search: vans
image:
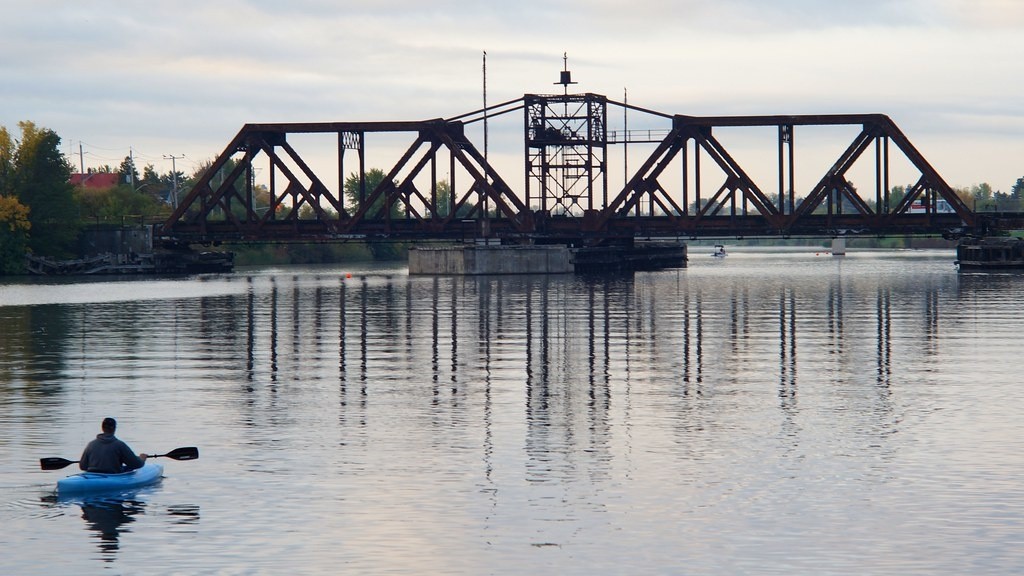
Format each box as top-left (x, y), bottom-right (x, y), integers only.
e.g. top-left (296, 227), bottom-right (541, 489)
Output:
top-left (908, 200), bottom-right (956, 213)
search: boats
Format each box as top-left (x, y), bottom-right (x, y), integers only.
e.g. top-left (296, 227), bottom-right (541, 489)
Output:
top-left (714, 244), bottom-right (727, 258)
top-left (56, 459), bottom-right (165, 500)
top-left (197, 250), bottom-right (235, 273)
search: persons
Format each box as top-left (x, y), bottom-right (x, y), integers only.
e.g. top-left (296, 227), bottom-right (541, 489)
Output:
top-left (79, 418), bottom-right (147, 474)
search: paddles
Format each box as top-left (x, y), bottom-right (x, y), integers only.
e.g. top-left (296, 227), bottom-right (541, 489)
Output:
top-left (39, 446), bottom-right (200, 471)
